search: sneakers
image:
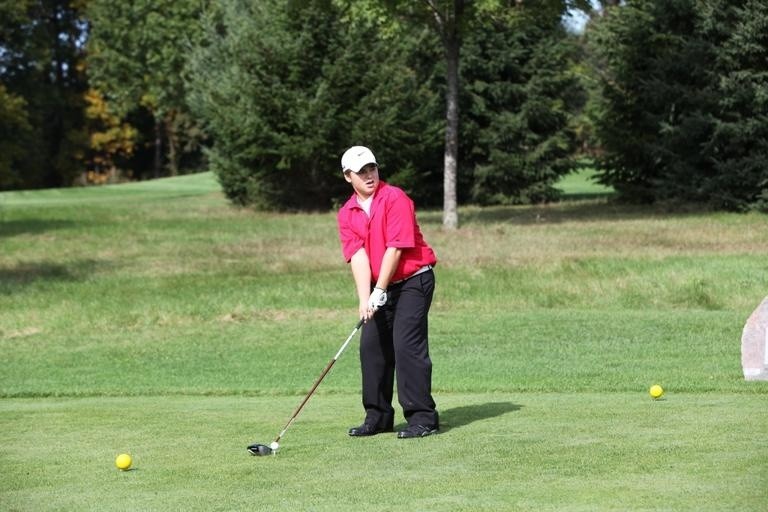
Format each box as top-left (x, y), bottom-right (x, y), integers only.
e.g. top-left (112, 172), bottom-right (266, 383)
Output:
top-left (397, 424), bottom-right (439, 439)
top-left (349, 423), bottom-right (394, 436)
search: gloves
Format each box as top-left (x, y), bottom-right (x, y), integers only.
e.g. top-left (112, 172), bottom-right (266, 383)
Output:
top-left (368, 288), bottom-right (384, 310)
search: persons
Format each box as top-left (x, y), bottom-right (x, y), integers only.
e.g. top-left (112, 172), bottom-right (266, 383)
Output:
top-left (336, 144), bottom-right (441, 439)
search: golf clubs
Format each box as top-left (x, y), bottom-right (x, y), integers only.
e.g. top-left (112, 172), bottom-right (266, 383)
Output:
top-left (246, 290), bottom-right (387, 456)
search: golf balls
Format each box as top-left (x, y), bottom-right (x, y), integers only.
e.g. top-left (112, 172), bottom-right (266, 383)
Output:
top-left (270, 441), bottom-right (279, 450)
top-left (649, 385), bottom-right (663, 400)
top-left (116, 453), bottom-right (132, 470)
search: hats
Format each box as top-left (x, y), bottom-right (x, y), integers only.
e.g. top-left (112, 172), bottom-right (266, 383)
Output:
top-left (341, 146), bottom-right (379, 173)
